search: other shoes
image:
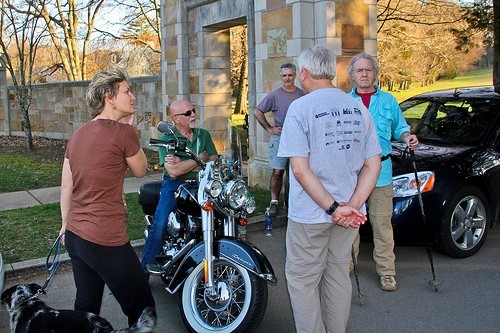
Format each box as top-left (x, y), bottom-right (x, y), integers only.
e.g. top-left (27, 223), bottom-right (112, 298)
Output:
top-left (379, 274), bottom-right (398, 292)
top-left (269, 200), bottom-right (279, 215)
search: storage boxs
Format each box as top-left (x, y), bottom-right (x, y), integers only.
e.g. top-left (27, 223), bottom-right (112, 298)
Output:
top-left (139, 181), bottom-right (161, 215)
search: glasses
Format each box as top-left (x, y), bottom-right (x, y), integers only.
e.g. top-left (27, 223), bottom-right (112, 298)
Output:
top-left (172, 108), bottom-right (197, 119)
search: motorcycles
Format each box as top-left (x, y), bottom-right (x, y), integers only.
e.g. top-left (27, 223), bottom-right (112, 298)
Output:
top-left (138, 121), bottom-right (278, 333)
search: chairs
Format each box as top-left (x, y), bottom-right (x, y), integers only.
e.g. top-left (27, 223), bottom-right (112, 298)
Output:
top-left (434, 108), bottom-right (471, 138)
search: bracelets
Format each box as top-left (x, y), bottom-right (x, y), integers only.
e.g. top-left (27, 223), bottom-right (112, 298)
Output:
top-left (325, 201), bottom-right (339, 215)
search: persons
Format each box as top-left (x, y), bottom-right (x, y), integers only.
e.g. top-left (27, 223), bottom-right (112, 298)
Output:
top-left (59, 72), bottom-right (156, 333)
top-left (230, 63), bottom-right (306, 217)
top-left (277, 45), bottom-right (382, 333)
top-left (140, 100), bottom-right (219, 277)
top-left (347, 54), bottom-right (418, 291)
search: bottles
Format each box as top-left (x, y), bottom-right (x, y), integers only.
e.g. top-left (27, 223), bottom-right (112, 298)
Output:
top-left (265, 207), bottom-right (273, 237)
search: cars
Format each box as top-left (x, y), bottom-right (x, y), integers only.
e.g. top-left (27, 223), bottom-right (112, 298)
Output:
top-left (358, 85), bottom-right (500, 260)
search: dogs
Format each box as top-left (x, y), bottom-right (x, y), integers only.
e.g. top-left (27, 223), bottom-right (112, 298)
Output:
top-left (0, 283), bottom-right (155, 333)
